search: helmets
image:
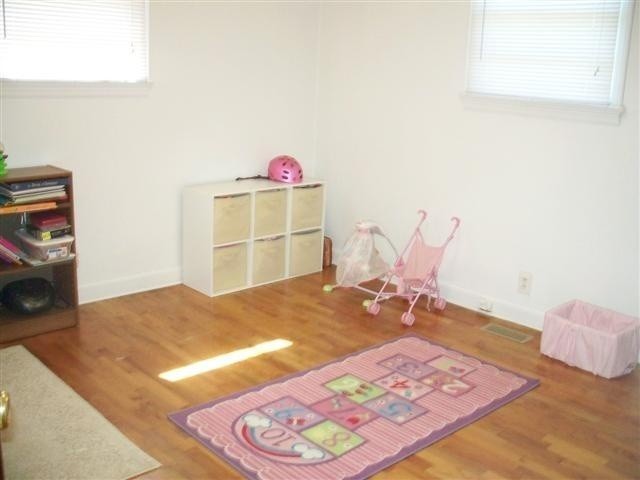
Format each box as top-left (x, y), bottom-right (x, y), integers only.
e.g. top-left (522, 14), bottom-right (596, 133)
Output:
top-left (268, 156), bottom-right (303, 183)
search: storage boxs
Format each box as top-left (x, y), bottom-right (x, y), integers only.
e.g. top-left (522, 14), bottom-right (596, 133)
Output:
top-left (538, 297), bottom-right (640, 381)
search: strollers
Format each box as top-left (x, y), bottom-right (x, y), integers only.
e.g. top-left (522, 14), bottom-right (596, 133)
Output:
top-left (366, 209), bottom-right (461, 327)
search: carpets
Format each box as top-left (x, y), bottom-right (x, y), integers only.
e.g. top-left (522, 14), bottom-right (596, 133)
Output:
top-left (0, 344), bottom-right (164, 480)
top-left (166, 331), bottom-right (543, 479)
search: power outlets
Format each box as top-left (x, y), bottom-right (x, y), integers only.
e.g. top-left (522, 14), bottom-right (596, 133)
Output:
top-left (515, 269), bottom-right (534, 298)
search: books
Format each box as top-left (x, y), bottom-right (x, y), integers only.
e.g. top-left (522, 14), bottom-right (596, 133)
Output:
top-left (1, 177), bottom-right (75, 265)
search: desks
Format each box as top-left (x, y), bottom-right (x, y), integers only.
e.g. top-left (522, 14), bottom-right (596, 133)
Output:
top-left (180, 177), bottom-right (327, 298)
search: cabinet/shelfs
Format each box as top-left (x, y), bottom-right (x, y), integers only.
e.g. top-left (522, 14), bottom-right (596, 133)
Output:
top-left (0, 163), bottom-right (86, 346)
